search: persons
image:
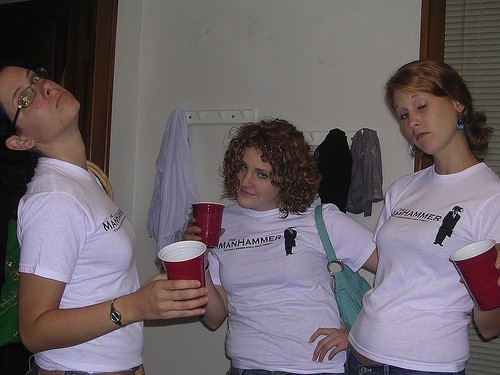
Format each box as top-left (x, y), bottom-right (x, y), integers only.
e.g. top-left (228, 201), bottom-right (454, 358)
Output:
top-left (0, 65), bottom-right (209, 374)
top-left (344, 60), bottom-right (500, 375)
top-left (182, 118), bottom-right (381, 375)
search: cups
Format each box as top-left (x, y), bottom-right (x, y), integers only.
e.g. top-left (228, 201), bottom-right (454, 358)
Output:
top-left (191, 201), bottom-right (226, 249)
top-left (449, 239), bottom-right (500, 310)
top-left (157, 240), bottom-right (206, 309)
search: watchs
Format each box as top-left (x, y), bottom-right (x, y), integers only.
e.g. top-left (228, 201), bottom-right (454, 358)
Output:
top-left (110, 297), bottom-right (125, 328)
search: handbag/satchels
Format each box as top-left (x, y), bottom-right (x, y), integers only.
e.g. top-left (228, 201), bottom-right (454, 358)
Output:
top-left (315, 204), bottom-right (373, 330)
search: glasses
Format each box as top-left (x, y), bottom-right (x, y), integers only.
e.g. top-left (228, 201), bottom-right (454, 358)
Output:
top-left (13, 66), bottom-right (47, 125)
